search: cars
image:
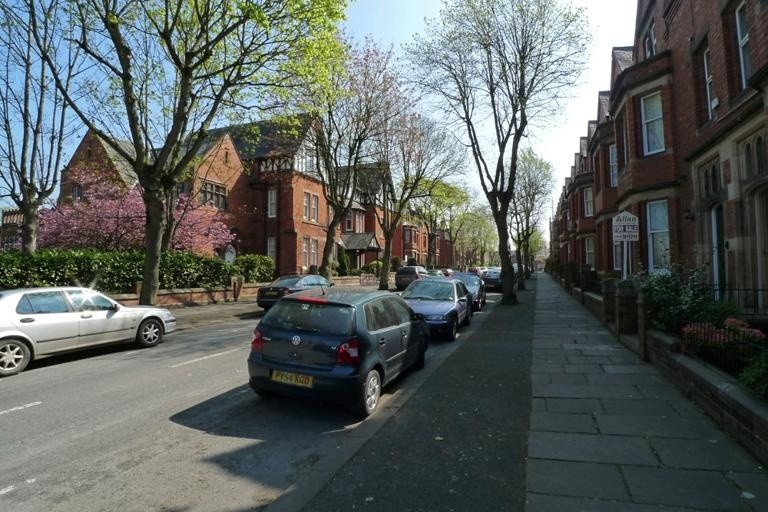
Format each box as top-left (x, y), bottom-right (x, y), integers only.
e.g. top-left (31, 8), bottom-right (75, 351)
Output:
top-left (471, 263), bottom-right (526, 290)
top-left (246, 288), bottom-right (430, 415)
top-left (0, 285), bottom-right (176, 377)
top-left (449, 272), bottom-right (486, 311)
top-left (256, 274), bottom-right (327, 311)
top-left (402, 277), bottom-right (473, 341)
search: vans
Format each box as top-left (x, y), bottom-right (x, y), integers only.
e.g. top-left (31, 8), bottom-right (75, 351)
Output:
top-left (394, 265), bottom-right (451, 288)
